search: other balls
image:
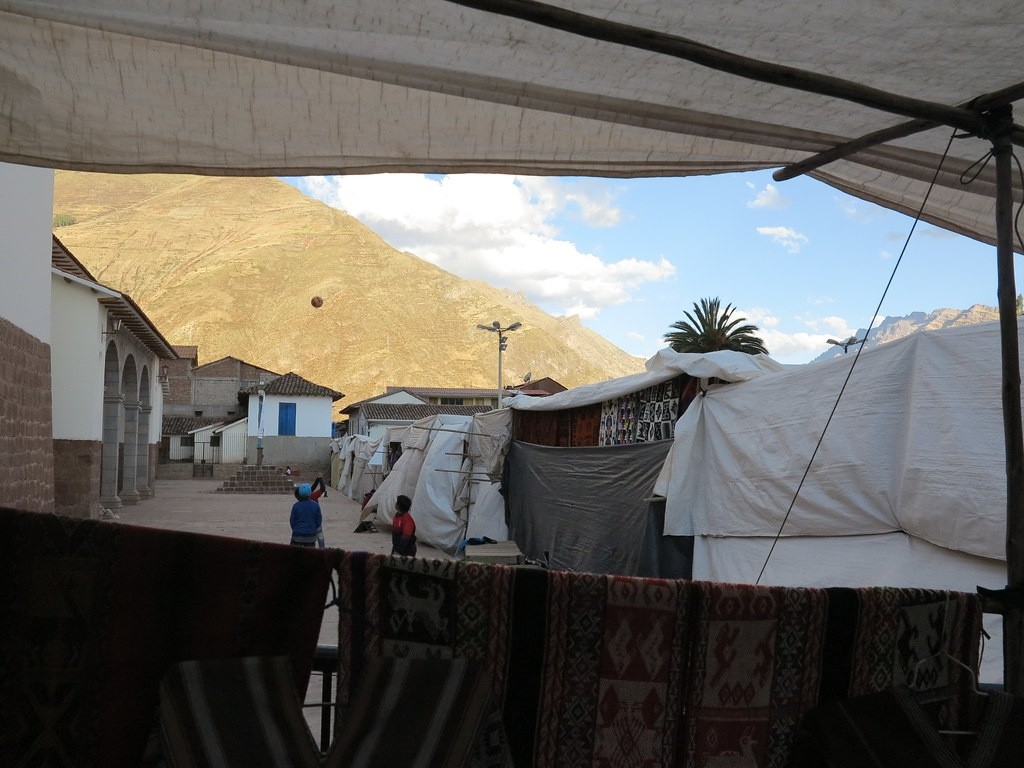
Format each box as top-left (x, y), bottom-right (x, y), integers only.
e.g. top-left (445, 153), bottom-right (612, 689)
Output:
top-left (311, 297), bottom-right (323, 309)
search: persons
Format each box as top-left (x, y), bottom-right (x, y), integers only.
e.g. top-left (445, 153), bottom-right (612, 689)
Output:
top-left (391, 495), bottom-right (417, 557)
top-left (289, 472), bottom-right (327, 548)
top-left (361, 489), bottom-right (378, 513)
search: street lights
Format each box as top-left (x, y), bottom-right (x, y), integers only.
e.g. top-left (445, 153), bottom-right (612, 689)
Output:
top-left (476, 320), bottom-right (522, 408)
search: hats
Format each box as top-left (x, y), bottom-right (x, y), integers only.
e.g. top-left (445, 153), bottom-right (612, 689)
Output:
top-left (299, 483), bottom-right (311, 497)
